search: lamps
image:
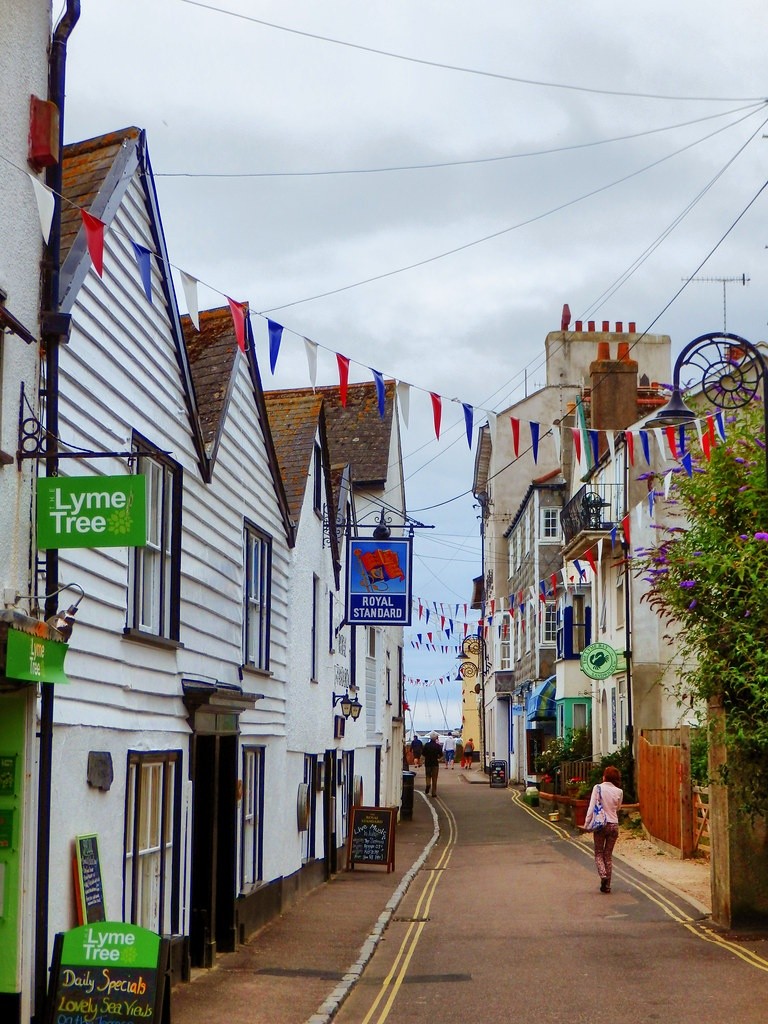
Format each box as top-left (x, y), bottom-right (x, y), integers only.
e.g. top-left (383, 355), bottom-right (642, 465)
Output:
top-left (519, 682), bottom-right (531, 697)
top-left (454, 662), bottom-right (478, 682)
top-left (3, 583), bottom-right (85, 639)
top-left (371, 509), bottom-right (391, 540)
top-left (333, 688), bottom-right (362, 722)
top-left (641, 330), bottom-right (768, 485)
top-left (455, 633), bottom-right (488, 661)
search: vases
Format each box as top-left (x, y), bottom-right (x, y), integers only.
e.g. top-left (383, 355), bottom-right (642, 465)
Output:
top-left (568, 785), bottom-right (579, 795)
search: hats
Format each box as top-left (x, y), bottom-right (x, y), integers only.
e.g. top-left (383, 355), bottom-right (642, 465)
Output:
top-left (430, 733), bottom-right (440, 740)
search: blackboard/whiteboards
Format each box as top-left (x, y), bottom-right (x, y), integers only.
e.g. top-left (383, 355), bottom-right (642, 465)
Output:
top-left (346, 805), bottom-right (396, 865)
top-left (76, 833), bottom-right (106, 925)
top-left (42, 922), bottom-right (173, 1024)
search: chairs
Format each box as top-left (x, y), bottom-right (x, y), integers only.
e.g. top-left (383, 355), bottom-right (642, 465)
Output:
top-left (580, 492), bottom-right (603, 529)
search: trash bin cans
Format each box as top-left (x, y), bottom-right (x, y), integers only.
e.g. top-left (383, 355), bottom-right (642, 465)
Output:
top-left (400, 771), bottom-right (416, 820)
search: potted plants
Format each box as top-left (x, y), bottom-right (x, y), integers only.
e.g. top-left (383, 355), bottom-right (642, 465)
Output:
top-left (575, 783), bottom-right (592, 799)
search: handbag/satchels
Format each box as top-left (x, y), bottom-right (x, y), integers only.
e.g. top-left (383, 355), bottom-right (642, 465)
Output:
top-left (461, 754), bottom-right (465, 767)
top-left (592, 784), bottom-right (607, 831)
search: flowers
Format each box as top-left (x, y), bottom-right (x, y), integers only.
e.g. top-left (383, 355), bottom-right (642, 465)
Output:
top-left (566, 776), bottom-right (587, 786)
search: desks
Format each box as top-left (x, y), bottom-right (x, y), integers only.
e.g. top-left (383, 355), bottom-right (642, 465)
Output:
top-left (590, 502), bottom-right (611, 527)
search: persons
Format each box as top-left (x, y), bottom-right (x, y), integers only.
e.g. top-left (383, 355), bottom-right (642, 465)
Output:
top-left (410, 736), bottom-right (423, 768)
top-left (583, 767), bottom-right (623, 894)
top-left (443, 732), bottom-right (457, 770)
top-left (463, 738), bottom-right (475, 770)
top-left (422, 732), bottom-right (443, 799)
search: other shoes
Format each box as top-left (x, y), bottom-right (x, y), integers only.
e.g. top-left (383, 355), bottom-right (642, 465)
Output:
top-left (600, 879), bottom-right (607, 892)
top-left (605, 888), bottom-right (610, 893)
top-left (432, 794), bottom-right (437, 798)
top-left (425, 785), bottom-right (431, 794)
